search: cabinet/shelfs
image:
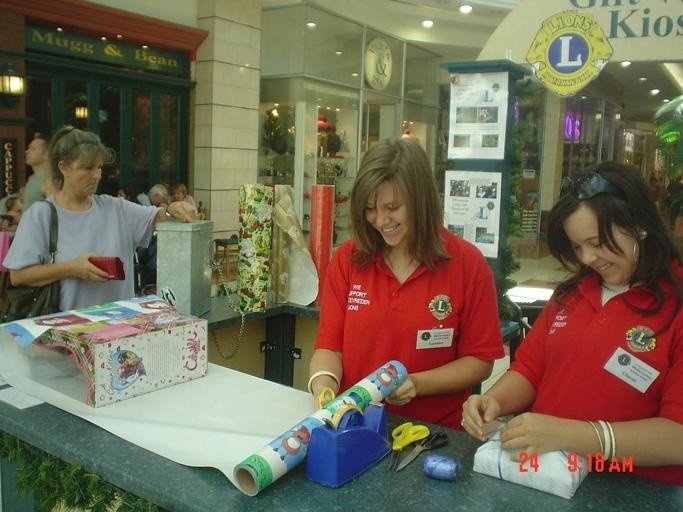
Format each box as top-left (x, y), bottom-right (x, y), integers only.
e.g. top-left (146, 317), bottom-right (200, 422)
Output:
top-left (506, 84), bottom-right (652, 260)
top-left (257, 75), bottom-right (442, 303)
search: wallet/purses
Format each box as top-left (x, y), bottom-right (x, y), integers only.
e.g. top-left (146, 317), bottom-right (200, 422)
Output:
top-left (88, 256), bottom-right (126, 280)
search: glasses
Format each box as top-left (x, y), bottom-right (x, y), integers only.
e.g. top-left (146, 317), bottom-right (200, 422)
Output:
top-left (560, 172), bottom-right (624, 200)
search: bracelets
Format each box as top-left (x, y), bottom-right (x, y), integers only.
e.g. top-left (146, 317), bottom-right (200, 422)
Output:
top-left (597, 419), bottom-right (610, 461)
top-left (606, 421), bottom-right (616, 462)
top-left (586, 419), bottom-right (605, 454)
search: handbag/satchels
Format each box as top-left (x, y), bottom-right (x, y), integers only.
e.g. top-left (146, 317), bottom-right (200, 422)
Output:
top-left (0, 200), bottom-right (59, 324)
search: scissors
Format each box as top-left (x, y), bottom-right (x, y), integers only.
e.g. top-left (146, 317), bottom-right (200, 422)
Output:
top-left (319, 388), bottom-right (335, 409)
top-left (386, 422), bottom-right (448, 472)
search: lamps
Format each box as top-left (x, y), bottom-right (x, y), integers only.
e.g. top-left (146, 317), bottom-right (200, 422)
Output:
top-left (0, 61), bottom-right (25, 109)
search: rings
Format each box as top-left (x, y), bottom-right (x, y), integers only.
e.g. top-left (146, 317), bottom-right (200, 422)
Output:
top-left (460, 418), bottom-right (465, 426)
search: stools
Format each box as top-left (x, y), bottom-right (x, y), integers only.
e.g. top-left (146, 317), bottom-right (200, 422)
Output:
top-left (214, 239), bottom-right (240, 281)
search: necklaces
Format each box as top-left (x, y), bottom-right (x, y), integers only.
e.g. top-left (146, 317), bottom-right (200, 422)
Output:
top-left (307, 370), bottom-right (340, 395)
top-left (598, 283), bottom-right (618, 294)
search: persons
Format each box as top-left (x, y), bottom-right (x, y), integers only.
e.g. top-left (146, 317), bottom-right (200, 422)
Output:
top-left (1, 125), bottom-right (199, 311)
top-left (20, 132), bottom-right (51, 213)
top-left (0, 182), bottom-right (199, 287)
top-left (461, 161), bottom-right (683, 487)
top-left (648, 175), bottom-right (683, 232)
top-left (306, 134), bottom-right (506, 431)
